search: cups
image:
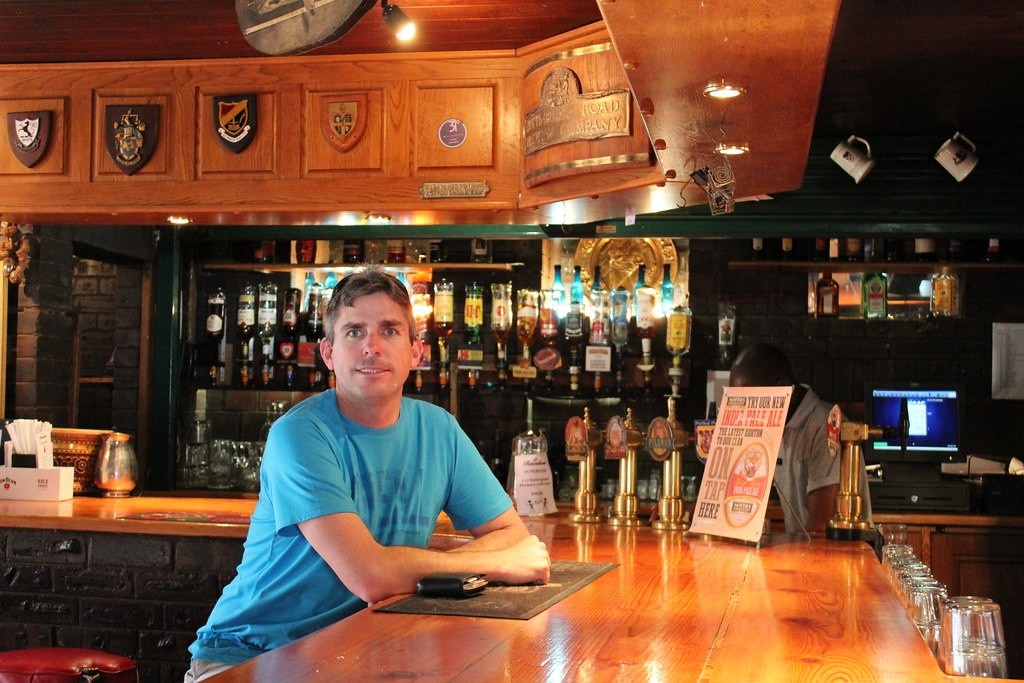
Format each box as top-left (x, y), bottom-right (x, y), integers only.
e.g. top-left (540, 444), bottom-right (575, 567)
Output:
top-left (174, 400), bottom-right (291, 492)
top-left (879, 523), bottom-right (1009, 679)
top-left (830, 135), bottom-right (878, 183)
top-left (934, 131), bottom-right (979, 182)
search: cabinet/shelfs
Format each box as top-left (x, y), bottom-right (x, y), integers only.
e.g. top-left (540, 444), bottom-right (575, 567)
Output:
top-left (923, 516), bottom-right (1024, 646)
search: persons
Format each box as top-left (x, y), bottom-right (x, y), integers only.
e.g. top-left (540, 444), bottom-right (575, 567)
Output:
top-left (184, 271), bottom-right (551, 683)
top-left (731, 346), bottom-right (874, 534)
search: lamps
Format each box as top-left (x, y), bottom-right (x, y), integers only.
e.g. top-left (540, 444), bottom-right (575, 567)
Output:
top-left (381, 1), bottom-right (418, 43)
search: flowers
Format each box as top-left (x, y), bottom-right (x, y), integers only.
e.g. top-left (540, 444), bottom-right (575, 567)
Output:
top-left (0, 223), bottom-right (34, 284)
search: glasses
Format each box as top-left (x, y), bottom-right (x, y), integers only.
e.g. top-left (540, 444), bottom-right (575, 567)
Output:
top-left (331, 272), bottom-right (408, 295)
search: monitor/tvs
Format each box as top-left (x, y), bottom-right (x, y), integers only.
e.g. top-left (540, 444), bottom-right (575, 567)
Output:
top-left (870, 387), bottom-right (966, 464)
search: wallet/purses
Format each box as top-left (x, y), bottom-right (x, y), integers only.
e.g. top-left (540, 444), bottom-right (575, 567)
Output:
top-left (417, 571), bottom-right (487, 598)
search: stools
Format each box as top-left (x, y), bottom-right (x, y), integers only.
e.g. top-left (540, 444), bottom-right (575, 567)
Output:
top-left (1, 649), bottom-right (140, 683)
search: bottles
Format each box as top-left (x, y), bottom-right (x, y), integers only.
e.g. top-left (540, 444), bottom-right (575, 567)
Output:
top-left (206, 240), bottom-right (692, 394)
top-left (716, 295), bottom-right (737, 371)
top-left (489, 428), bottom-right (505, 487)
top-left (601, 478), bottom-right (617, 499)
top-left (752, 238), bottom-right (1003, 319)
top-left (680, 475), bottom-right (697, 502)
top-left (648, 468), bottom-right (662, 501)
